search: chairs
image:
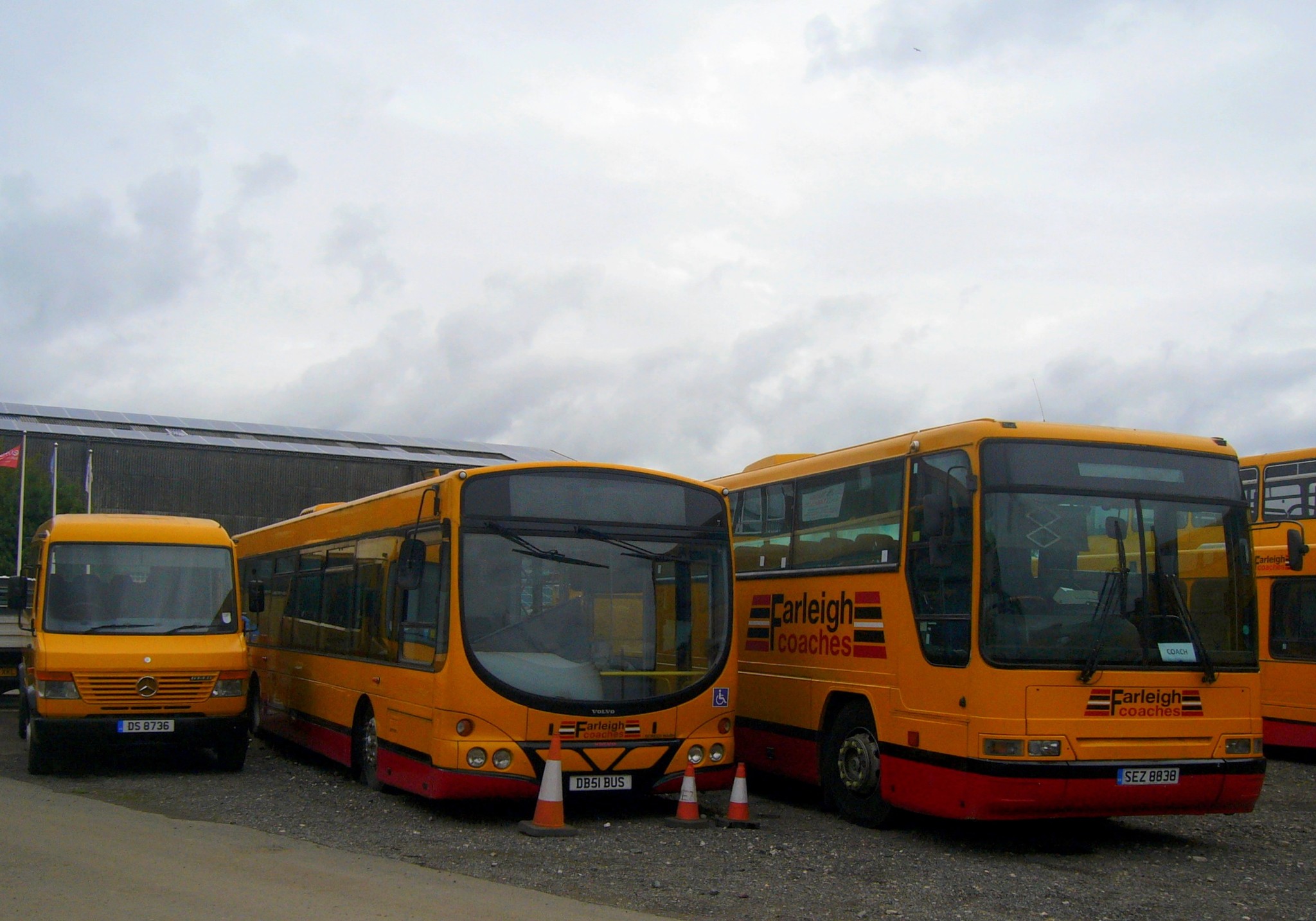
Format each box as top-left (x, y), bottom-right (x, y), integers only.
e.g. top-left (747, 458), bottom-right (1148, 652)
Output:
top-left (734, 515), bottom-right (1098, 657)
top-left (48, 566), bottom-right (204, 621)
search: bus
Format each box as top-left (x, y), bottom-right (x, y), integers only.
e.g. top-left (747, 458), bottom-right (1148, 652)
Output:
top-left (1020, 446), bottom-right (1316, 749)
top-left (230, 462), bottom-right (739, 809)
top-left (704, 419), bottom-right (1265, 843)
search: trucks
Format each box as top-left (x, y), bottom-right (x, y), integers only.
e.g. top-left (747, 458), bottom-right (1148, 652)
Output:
top-left (0, 575), bottom-right (39, 710)
top-left (6, 512), bottom-right (268, 778)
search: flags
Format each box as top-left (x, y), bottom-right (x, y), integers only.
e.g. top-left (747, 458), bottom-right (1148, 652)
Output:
top-left (0, 442), bottom-right (21, 470)
top-left (50, 453), bottom-right (55, 484)
top-left (85, 457), bottom-right (94, 492)
